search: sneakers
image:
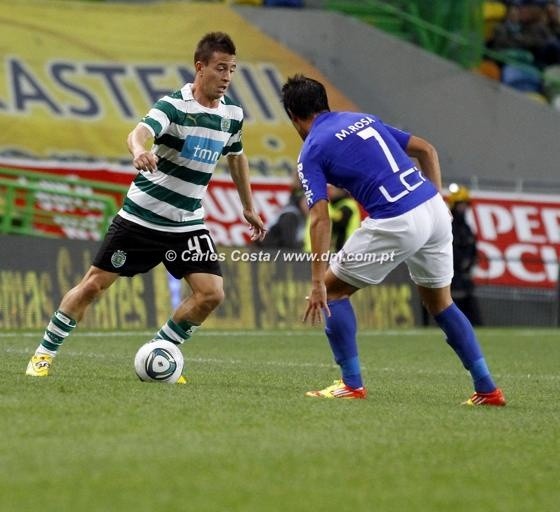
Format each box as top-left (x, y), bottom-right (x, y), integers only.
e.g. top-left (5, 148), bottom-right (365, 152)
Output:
top-left (304, 377), bottom-right (365, 398)
top-left (25, 351), bottom-right (55, 378)
top-left (461, 389), bottom-right (508, 408)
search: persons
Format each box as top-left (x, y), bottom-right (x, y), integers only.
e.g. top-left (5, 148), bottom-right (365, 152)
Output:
top-left (256, 176), bottom-right (485, 327)
top-left (24, 32), bottom-right (269, 384)
top-left (474, 0), bottom-right (560, 110)
top-left (281, 75), bottom-right (505, 406)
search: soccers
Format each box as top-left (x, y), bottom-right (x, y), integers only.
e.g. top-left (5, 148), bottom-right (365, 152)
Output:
top-left (135, 339), bottom-right (184, 384)
